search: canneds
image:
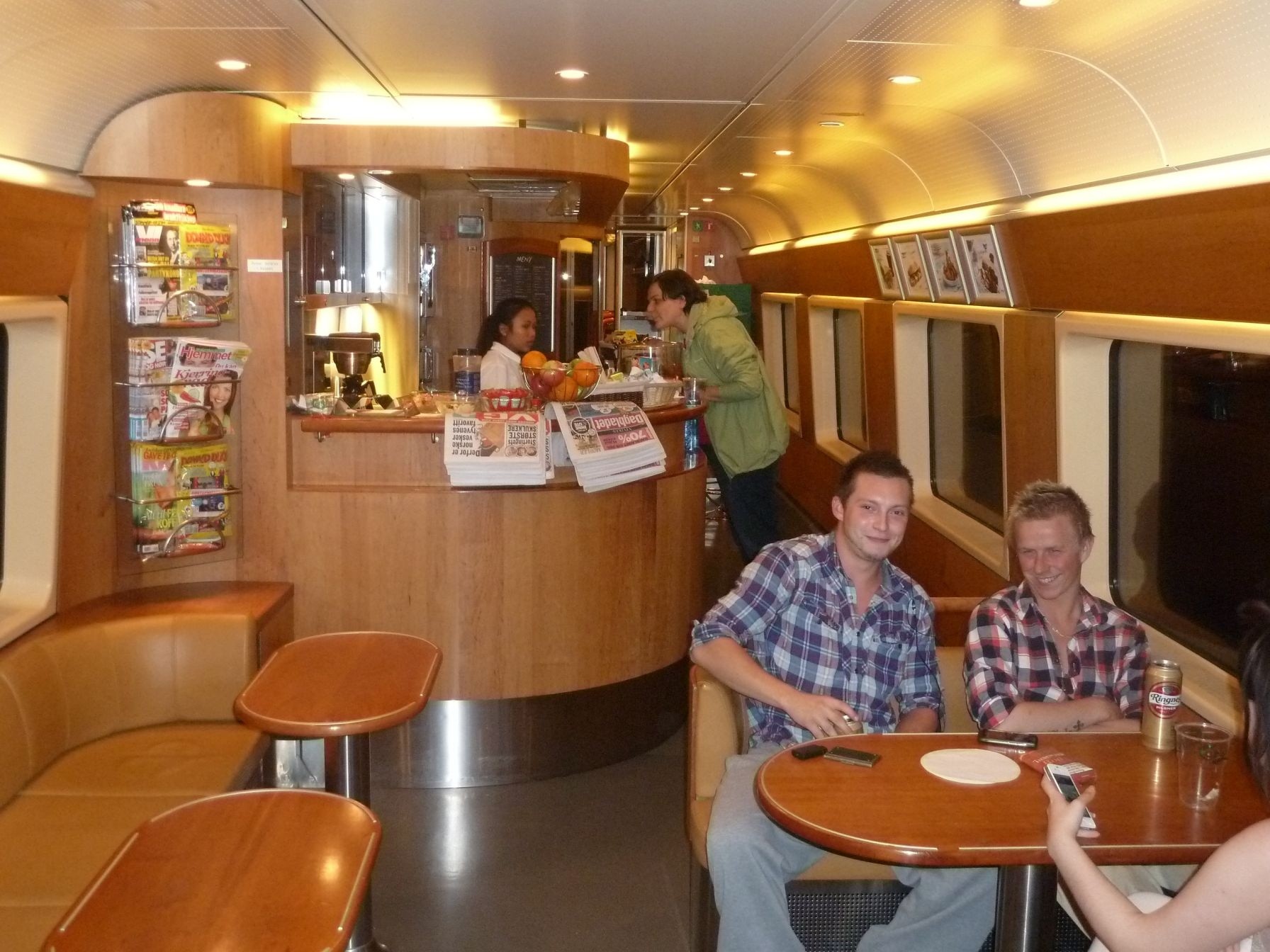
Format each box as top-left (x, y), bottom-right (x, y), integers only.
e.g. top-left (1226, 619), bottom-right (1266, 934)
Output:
top-left (1139, 658), bottom-right (1186, 754)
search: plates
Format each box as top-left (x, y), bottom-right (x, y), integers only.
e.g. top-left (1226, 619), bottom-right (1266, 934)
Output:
top-left (920, 748), bottom-right (1021, 785)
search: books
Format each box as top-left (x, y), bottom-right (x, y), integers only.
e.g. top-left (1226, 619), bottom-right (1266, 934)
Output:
top-left (122, 218), bottom-right (252, 558)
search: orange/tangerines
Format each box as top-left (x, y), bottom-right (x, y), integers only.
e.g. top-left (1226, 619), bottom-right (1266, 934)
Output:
top-left (522, 351), bottom-right (547, 369)
top-left (551, 377), bottom-right (579, 402)
top-left (573, 362), bottom-right (600, 387)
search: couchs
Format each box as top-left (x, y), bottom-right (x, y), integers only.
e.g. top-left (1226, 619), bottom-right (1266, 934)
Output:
top-left (684, 594), bottom-right (988, 952)
top-left (0, 579), bottom-right (295, 952)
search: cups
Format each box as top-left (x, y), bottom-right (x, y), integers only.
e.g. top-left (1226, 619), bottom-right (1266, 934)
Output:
top-left (1175, 723), bottom-right (1231, 805)
top-left (681, 378), bottom-right (707, 407)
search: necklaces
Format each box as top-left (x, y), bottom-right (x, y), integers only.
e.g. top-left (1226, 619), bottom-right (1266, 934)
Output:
top-left (1038, 608), bottom-right (1075, 639)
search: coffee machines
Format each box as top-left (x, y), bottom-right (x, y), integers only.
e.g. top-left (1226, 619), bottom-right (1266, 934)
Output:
top-left (304, 330), bottom-right (385, 399)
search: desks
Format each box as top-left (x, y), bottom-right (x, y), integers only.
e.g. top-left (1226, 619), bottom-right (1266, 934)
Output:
top-left (753, 732), bottom-right (1270, 952)
top-left (39, 787), bottom-right (383, 952)
top-left (232, 629), bottom-right (442, 952)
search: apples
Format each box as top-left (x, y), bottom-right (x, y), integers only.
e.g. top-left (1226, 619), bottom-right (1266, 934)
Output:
top-left (540, 360), bottom-right (567, 386)
top-left (529, 376), bottom-right (552, 400)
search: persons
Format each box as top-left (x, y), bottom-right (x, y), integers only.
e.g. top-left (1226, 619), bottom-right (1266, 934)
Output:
top-left (647, 268), bottom-right (789, 564)
top-left (1038, 604), bottom-right (1270, 951)
top-left (477, 297), bottom-right (537, 391)
top-left (962, 481), bottom-right (1200, 944)
top-left (691, 448), bottom-right (998, 952)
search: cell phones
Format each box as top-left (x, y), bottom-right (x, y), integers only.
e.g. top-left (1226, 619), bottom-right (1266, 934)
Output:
top-left (824, 746), bottom-right (881, 767)
top-left (1043, 764), bottom-right (1097, 829)
top-left (977, 729), bottom-right (1039, 748)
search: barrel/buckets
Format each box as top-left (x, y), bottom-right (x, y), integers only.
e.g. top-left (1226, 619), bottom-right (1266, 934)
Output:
top-left (451, 348), bottom-right (482, 395)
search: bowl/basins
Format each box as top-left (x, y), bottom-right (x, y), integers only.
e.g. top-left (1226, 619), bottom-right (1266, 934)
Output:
top-left (482, 397), bottom-right (526, 411)
top-left (519, 362), bottom-right (602, 403)
top-left (433, 394), bottom-right (480, 416)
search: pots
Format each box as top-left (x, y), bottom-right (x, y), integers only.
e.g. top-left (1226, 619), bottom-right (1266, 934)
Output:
top-left (611, 336), bottom-right (683, 380)
